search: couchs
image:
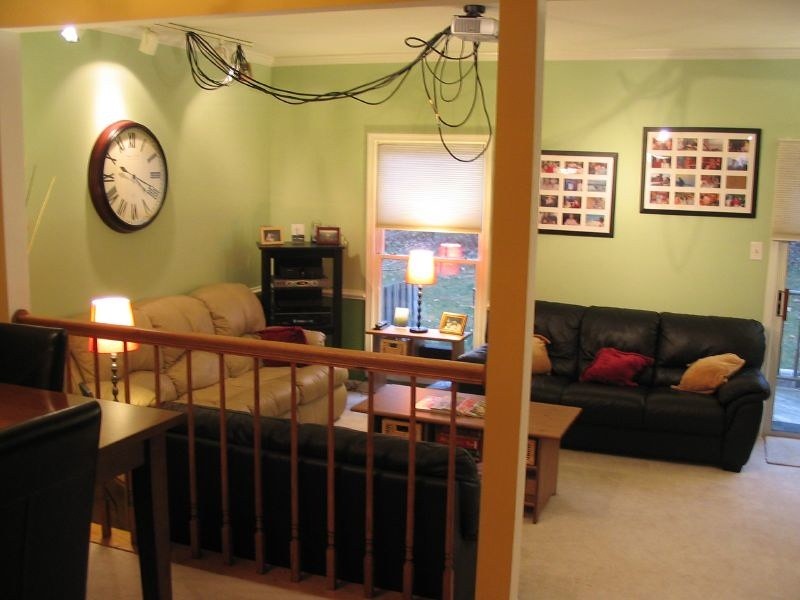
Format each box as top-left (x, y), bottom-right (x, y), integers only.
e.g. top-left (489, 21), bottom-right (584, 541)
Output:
top-left (456, 300), bottom-right (772, 474)
top-left (60, 279), bottom-right (351, 531)
top-left (132, 400), bottom-right (480, 600)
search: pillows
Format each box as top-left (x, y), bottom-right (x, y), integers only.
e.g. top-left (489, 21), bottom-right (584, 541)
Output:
top-left (578, 346), bottom-right (655, 388)
top-left (671, 352), bottom-right (745, 394)
top-left (254, 326), bottom-right (311, 368)
top-left (531, 334), bottom-right (552, 377)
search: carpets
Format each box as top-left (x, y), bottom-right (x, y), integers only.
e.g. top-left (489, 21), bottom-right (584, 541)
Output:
top-left (763, 435), bottom-right (800, 468)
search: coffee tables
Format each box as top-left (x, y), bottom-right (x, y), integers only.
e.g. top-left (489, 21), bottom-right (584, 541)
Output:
top-left (351, 384), bottom-right (582, 525)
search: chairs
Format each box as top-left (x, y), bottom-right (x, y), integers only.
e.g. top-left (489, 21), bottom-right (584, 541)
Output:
top-left (0, 322), bottom-right (68, 393)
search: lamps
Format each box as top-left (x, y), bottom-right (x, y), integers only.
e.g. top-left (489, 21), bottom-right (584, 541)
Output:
top-left (136, 24), bottom-right (160, 57)
top-left (88, 295), bottom-right (139, 402)
top-left (405, 248), bottom-right (437, 334)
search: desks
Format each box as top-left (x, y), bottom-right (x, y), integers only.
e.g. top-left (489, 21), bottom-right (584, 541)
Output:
top-left (364, 325), bottom-right (473, 361)
top-left (0, 384), bottom-right (103, 600)
top-left (98, 399), bottom-right (186, 600)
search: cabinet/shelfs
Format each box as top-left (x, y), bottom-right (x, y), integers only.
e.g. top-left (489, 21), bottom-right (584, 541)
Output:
top-left (255, 241), bottom-right (349, 348)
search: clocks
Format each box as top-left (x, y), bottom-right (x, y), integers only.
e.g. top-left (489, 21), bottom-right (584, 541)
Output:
top-left (88, 119), bottom-right (169, 234)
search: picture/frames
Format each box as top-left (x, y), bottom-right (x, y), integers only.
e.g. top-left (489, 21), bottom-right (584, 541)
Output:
top-left (315, 226), bottom-right (341, 245)
top-left (538, 150), bottom-right (618, 239)
top-left (260, 226), bottom-right (286, 245)
top-left (438, 312), bottom-right (468, 336)
top-left (639, 125), bottom-right (762, 219)
